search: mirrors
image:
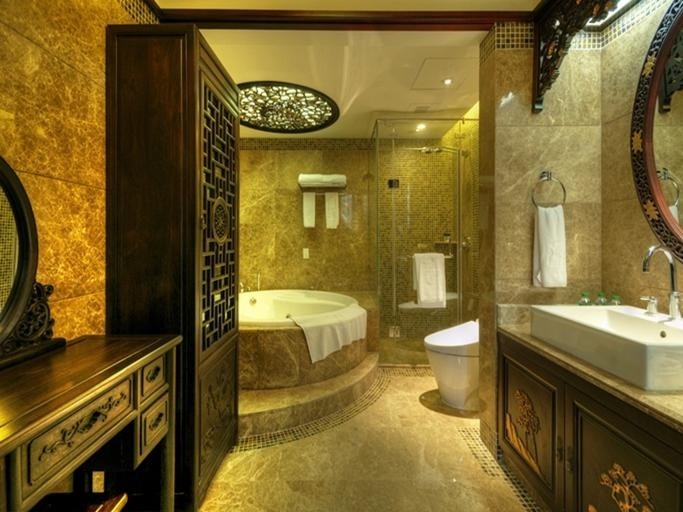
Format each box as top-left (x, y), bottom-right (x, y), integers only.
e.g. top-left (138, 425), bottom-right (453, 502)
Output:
top-left (0, 153), bottom-right (67, 370)
top-left (628, 0), bottom-right (683, 264)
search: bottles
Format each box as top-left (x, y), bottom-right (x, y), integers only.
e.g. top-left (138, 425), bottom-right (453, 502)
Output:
top-left (595, 291), bottom-right (609, 304)
top-left (578, 290), bottom-right (591, 306)
top-left (612, 294), bottom-right (621, 306)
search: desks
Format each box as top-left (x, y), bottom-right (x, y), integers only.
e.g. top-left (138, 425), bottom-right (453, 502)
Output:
top-left (1, 334), bottom-right (184, 512)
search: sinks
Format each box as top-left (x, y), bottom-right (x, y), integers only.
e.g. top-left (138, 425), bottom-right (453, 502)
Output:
top-left (529, 304), bottom-right (683, 392)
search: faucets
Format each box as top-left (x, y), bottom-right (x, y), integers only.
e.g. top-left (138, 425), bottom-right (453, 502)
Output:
top-left (642, 244), bottom-right (680, 319)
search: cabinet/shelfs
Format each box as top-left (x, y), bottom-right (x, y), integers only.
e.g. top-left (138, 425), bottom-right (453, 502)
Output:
top-left (495, 326), bottom-right (683, 512)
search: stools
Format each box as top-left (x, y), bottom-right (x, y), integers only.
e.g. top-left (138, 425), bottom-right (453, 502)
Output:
top-left (30, 490), bottom-right (128, 511)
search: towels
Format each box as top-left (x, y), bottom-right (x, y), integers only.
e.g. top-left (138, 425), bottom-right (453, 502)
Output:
top-left (285, 302), bottom-right (368, 364)
top-left (532, 203), bottom-right (568, 290)
top-left (411, 251), bottom-right (448, 309)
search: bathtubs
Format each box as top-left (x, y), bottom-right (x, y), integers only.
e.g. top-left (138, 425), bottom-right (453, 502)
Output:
top-left (239, 288), bottom-right (358, 326)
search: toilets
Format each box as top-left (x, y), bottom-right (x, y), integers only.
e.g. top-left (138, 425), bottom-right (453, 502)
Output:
top-left (424, 319), bottom-right (480, 411)
top-left (398, 292), bottom-right (459, 320)
